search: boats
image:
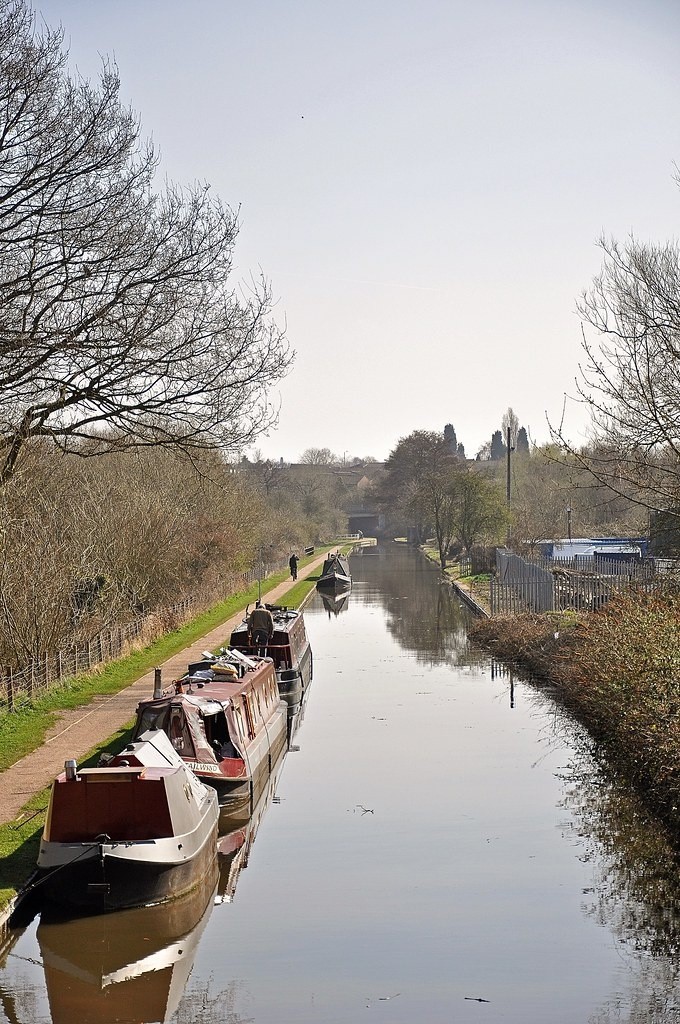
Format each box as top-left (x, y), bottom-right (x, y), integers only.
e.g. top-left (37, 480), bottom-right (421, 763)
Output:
top-left (221, 607), bottom-right (313, 707)
top-left (317, 586), bottom-right (352, 620)
top-left (33, 721), bottom-right (222, 910)
top-left (127, 647), bottom-right (288, 808)
top-left (316, 548), bottom-right (352, 587)
top-left (31, 826), bottom-right (220, 1024)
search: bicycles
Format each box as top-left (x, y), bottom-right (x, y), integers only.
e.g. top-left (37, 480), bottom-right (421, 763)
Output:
top-left (291, 566), bottom-right (297, 581)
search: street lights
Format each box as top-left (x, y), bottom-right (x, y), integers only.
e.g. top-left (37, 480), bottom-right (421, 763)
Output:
top-left (567, 505), bottom-right (572, 539)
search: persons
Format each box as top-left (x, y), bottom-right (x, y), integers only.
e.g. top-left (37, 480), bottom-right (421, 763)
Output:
top-left (289, 553), bottom-right (299, 580)
top-left (357, 529), bottom-right (363, 538)
top-left (247, 603), bottom-right (274, 659)
